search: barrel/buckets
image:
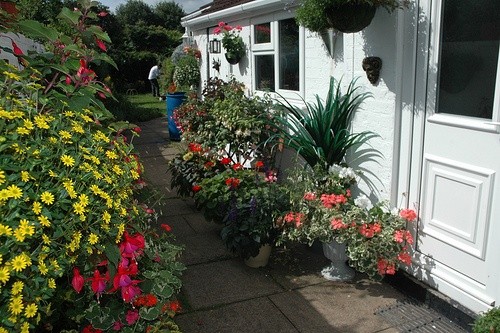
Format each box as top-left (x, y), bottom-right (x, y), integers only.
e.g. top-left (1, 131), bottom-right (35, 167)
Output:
top-left (166, 92), bottom-right (188, 137)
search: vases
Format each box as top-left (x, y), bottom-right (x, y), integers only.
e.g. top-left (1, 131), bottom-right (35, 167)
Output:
top-left (304, 240), bottom-right (321, 261)
top-left (326, 5), bottom-right (376, 33)
top-left (321, 241), bottom-right (355, 281)
top-left (245, 243), bottom-right (272, 268)
top-left (226, 53), bottom-right (241, 64)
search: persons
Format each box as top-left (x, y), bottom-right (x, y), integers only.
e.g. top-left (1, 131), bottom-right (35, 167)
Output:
top-left (148, 63), bottom-right (162, 97)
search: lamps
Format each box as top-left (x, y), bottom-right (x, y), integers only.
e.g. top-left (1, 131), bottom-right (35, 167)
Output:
top-left (210, 39), bottom-right (221, 53)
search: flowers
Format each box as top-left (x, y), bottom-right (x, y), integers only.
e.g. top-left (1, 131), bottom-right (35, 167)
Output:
top-left (279, 0), bottom-right (410, 32)
top-left (213, 21), bottom-right (246, 58)
top-left (164, 77), bottom-right (417, 282)
